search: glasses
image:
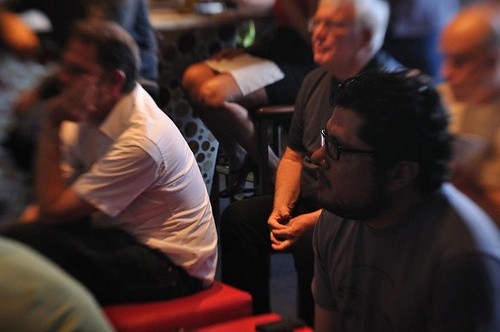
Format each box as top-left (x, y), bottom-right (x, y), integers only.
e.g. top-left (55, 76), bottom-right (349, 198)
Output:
top-left (320, 129), bottom-right (374, 162)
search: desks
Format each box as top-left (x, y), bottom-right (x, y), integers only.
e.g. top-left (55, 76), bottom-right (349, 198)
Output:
top-left (146, 7), bottom-right (212, 43)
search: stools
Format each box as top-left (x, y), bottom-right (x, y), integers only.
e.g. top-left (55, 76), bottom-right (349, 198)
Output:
top-left (101, 281), bottom-right (314, 332)
top-left (229, 103), bottom-right (295, 201)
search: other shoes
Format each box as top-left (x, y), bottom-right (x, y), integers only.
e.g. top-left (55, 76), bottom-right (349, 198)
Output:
top-left (230, 189), bottom-right (256, 203)
top-left (219, 179), bottom-right (245, 197)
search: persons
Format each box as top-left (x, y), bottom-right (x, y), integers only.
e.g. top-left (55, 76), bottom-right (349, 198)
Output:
top-left (0, 20), bottom-right (218, 307)
top-left (443, 7), bottom-right (500, 229)
top-left (311, 70), bottom-right (500, 332)
top-left (217, 0), bottom-right (406, 332)
top-left (0, 238), bottom-right (113, 332)
top-left (181, 0), bottom-right (319, 198)
top-left (0, 0), bottom-right (160, 118)
